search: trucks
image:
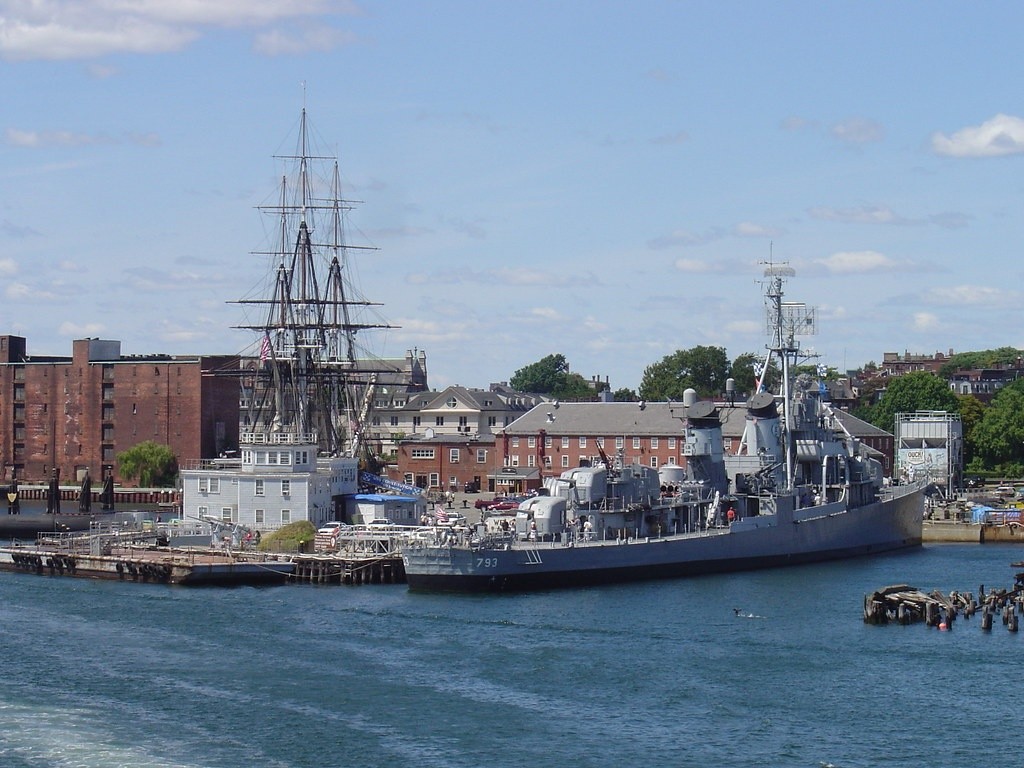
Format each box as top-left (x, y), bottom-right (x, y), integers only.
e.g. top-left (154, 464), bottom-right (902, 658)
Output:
top-left (318, 521), bottom-right (351, 536)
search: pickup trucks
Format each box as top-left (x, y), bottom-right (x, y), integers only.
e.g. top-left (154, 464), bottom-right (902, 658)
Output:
top-left (367, 518), bottom-right (395, 531)
top-left (474, 497), bottom-right (520, 512)
top-left (437, 512), bottom-right (467, 528)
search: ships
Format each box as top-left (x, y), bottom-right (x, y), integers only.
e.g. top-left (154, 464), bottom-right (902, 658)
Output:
top-left (397, 237), bottom-right (937, 591)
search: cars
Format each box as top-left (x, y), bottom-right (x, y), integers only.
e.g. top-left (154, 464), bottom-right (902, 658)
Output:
top-left (963, 475), bottom-right (985, 487)
top-left (475, 516), bottom-right (514, 531)
top-left (1015, 487), bottom-right (1024, 497)
top-left (464, 482), bottom-right (478, 493)
top-left (401, 526), bottom-right (444, 541)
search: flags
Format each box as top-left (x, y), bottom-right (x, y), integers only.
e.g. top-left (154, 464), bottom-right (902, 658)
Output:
top-left (434, 505), bottom-right (448, 522)
top-left (819, 379), bottom-right (827, 398)
top-left (753, 363), bottom-right (766, 393)
top-left (260, 335), bottom-right (273, 363)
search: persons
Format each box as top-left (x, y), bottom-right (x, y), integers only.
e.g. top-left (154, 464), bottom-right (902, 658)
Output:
top-left (445, 489), bottom-right (455, 501)
top-left (222, 536), bottom-right (233, 551)
top-left (727, 507), bottom-right (734, 526)
top-left (420, 511), bottom-right (434, 526)
top-left (499, 485), bottom-right (507, 496)
top-left (658, 512), bottom-right (665, 533)
top-left (568, 515), bottom-right (593, 541)
top-left (245, 530), bottom-right (261, 546)
top-left (803, 492), bottom-right (821, 507)
top-left (530, 522), bottom-right (537, 547)
top-left (501, 519), bottom-right (515, 536)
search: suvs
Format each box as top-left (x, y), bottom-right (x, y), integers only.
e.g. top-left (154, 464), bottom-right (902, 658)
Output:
top-left (340, 524), bottom-right (371, 536)
top-left (470, 530), bottom-right (513, 547)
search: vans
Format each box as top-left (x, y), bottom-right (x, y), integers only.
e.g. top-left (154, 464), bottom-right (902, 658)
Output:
top-left (995, 487), bottom-right (1014, 498)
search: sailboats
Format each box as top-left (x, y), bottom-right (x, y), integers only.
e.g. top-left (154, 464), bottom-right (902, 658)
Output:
top-left (199, 73), bottom-right (423, 497)
